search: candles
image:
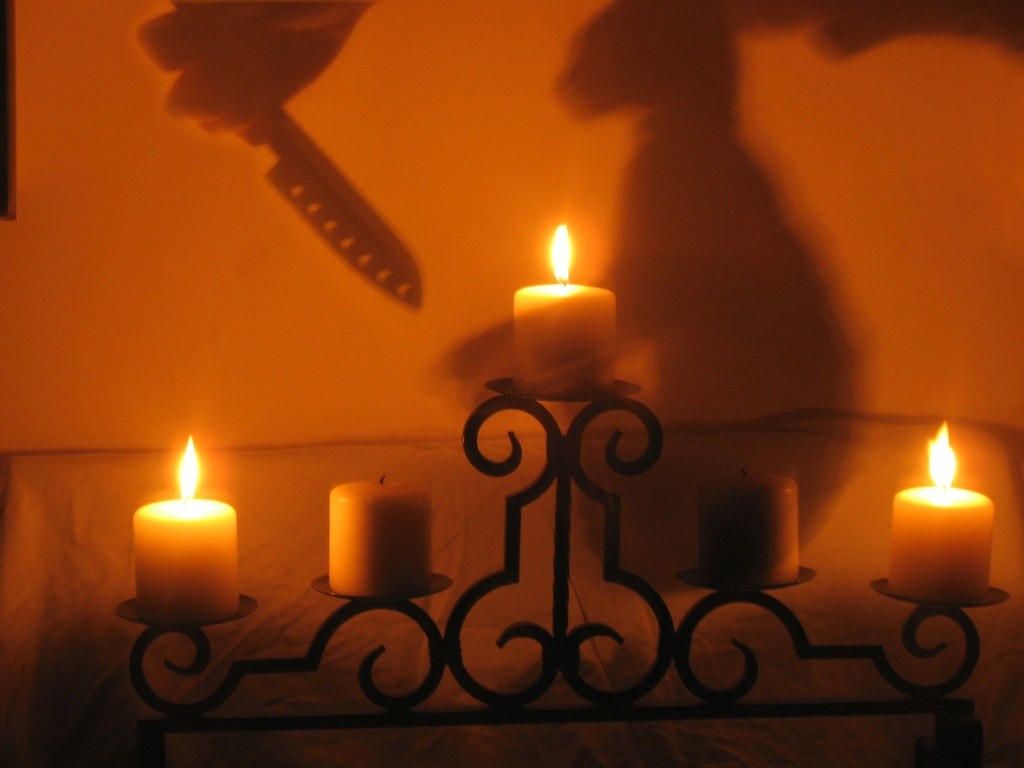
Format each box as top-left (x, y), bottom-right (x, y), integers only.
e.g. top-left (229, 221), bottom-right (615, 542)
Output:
top-left (512, 223), bottom-right (617, 397)
top-left (888, 420), bottom-right (995, 605)
top-left (699, 466), bottom-right (799, 588)
top-left (132, 435), bottom-right (238, 627)
top-left (329, 473), bottom-right (430, 598)
